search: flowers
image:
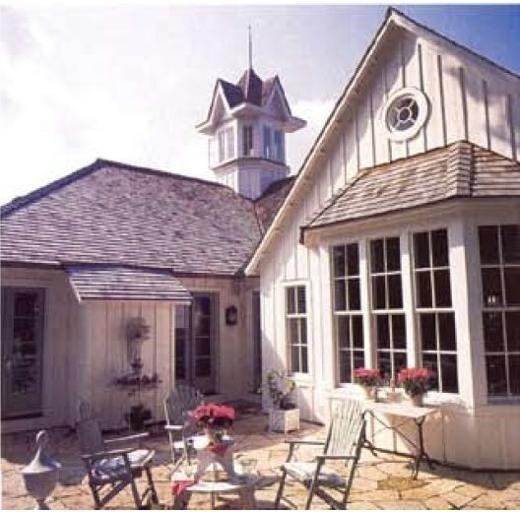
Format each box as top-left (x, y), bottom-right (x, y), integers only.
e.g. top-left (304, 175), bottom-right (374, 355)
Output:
top-left (353, 366), bottom-right (385, 387)
top-left (398, 367), bottom-right (436, 396)
top-left (181, 397), bottom-right (236, 430)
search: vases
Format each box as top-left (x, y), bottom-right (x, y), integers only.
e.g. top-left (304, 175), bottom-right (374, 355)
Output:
top-left (361, 386), bottom-right (372, 400)
top-left (408, 391), bottom-right (423, 406)
top-left (202, 425), bottom-right (226, 439)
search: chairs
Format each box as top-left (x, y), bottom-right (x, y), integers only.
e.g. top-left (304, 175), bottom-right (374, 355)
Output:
top-left (72, 396), bottom-right (160, 509)
top-left (160, 382), bottom-right (212, 465)
top-left (276, 398), bottom-right (370, 508)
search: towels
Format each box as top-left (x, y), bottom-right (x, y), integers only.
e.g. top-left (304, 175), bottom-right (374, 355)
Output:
top-left (173, 477), bottom-right (192, 496)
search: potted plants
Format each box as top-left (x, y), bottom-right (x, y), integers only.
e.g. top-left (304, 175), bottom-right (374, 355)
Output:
top-left (125, 316), bottom-right (151, 364)
top-left (257, 371), bottom-right (303, 433)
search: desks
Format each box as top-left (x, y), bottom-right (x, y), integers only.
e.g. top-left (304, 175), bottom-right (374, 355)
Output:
top-left (193, 434), bottom-right (240, 481)
top-left (327, 393), bottom-right (440, 479)
top-left (172, 461), bottom-right (281, 507)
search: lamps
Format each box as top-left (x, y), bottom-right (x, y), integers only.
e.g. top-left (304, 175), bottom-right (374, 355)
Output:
top-left (225, 305), bottom-right (238, 325)
